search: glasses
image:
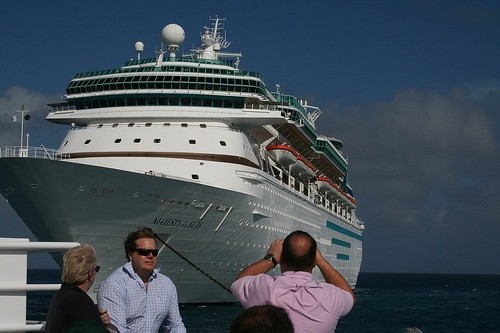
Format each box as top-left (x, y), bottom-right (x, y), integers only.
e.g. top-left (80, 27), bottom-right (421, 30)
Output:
top-left (133, 248), bottom-right (159, 257)
top-left (92, 266), bottom-right (100, 273)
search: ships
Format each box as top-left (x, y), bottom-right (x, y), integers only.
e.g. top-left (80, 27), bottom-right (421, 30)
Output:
top-left (0, 15), bottom-right (365, 305)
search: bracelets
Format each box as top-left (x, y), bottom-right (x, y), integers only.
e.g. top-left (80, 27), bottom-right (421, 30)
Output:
top-left (264, 253), bottom-right (277, 269)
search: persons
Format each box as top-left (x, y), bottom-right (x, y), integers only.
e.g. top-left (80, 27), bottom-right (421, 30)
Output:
top-left (46, 244), bottom-right (110, 333)
top-left (97, 228), bottom-right (187, 333)
top-left (231, 305), bottom-right (294, 333)
top-left (229, 230), bottom-right (357, 333)
